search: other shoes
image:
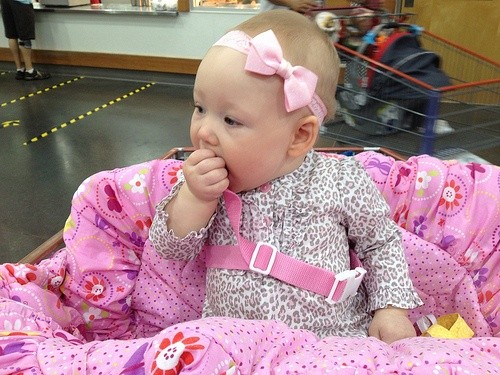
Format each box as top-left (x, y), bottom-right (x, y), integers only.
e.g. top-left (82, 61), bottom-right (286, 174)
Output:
top-left (25, 69), bottom-right (51, 80)
top-left (15, 69), bottom-right (26, 80)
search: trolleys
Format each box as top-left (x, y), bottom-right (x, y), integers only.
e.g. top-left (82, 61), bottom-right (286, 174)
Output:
top-left (302, 0), bottom-right (500, 157)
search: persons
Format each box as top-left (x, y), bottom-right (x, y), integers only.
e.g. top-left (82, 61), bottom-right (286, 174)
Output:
top-left (149, 9), bottom-right (424, 344)
top-left (0, 0), bottom-right (51, 80)
